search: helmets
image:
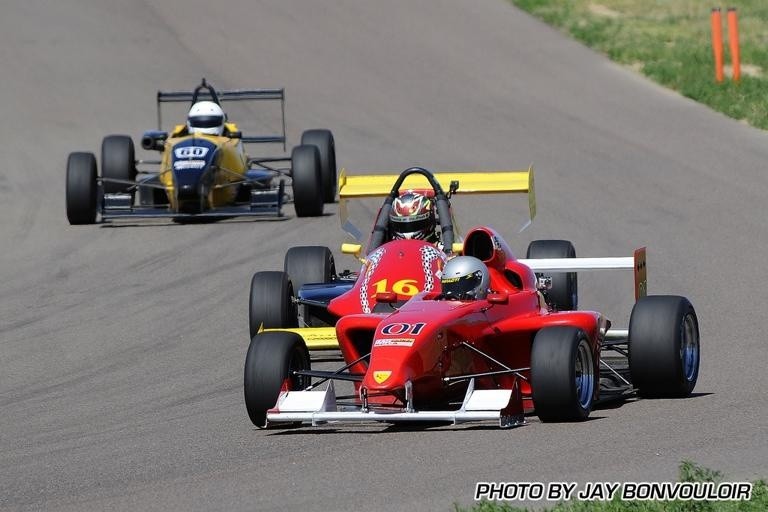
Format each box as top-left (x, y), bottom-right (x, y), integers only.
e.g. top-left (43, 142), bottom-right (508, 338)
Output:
top-left (442, 256), bottom-right (490, 302)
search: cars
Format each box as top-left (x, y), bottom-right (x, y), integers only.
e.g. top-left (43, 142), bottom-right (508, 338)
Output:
top-left (244, 225), bottom-right (702, 431)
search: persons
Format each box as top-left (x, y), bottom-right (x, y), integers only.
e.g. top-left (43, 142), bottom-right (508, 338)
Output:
top-left (434, 256), bottom-right (499, 300)
